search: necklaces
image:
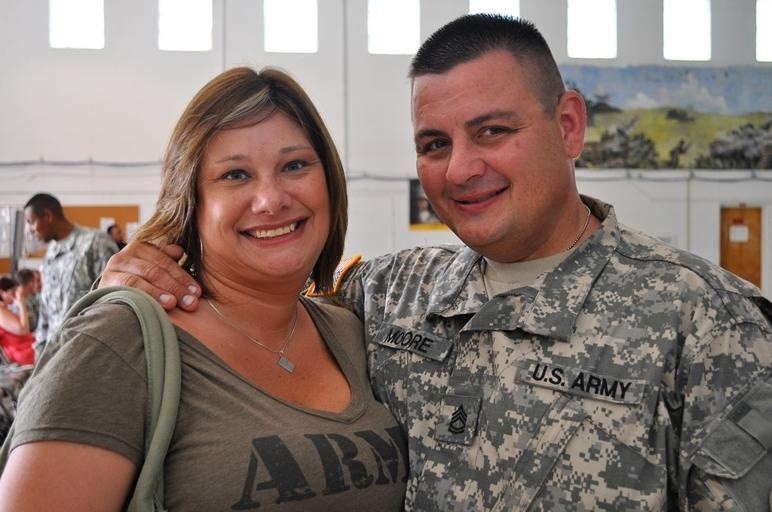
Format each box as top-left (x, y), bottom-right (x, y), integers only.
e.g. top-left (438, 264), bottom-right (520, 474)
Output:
top-left (566, 205), bottom-right (590, 252)
top-left (202, 292), bottom-right (300, 375)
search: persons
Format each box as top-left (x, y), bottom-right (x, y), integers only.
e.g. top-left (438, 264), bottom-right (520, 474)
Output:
top-left (1, 193), bottom-right (133, 368)
top-left (97, 14), bottom-right (771, 512)
top-left (2, 65), bottom-right (406, 511)
